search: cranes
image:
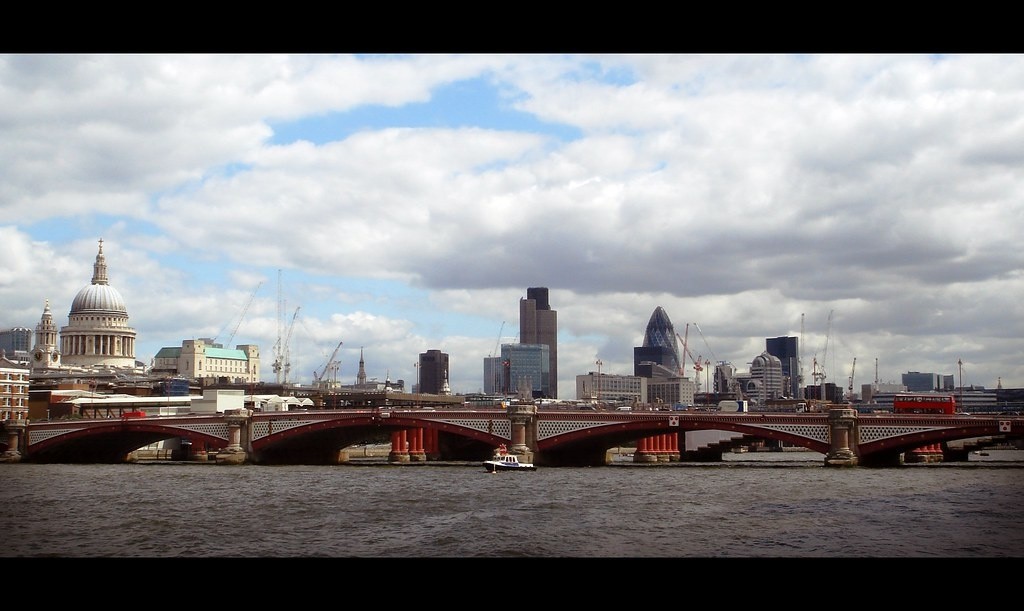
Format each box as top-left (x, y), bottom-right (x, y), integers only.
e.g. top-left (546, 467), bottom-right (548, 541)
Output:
top-left (800, 310), bottom-right (835, 385)
top-left (223, 268), bottom-right (345, 386)
top-left (676, 322), bottom-right (704, 377)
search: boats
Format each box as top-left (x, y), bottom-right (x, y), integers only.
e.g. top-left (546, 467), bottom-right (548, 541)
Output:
top-left (484, 454), bottom-right (540, 472)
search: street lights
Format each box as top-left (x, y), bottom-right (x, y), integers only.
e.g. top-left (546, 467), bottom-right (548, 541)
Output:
top-left (958, 358), bottom-right (964, 411)
top-left (88, 380), bottom-right (98, 418)
top-left (413, 361), bottom-right (421, 407)
top-left (501, 360), bottom-right (510, 400)
top-left (595, 359), bottom-right (603, 402)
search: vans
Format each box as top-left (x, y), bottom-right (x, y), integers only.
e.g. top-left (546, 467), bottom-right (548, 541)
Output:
top-left (120, 411), bottom-right (146, 418)
top-left (580, 406), bottom-right (595, 411)
top-left (616, 407), bottom-right (632, 411)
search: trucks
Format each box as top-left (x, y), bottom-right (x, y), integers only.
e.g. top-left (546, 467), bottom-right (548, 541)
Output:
top-left (714, 400), bottom-right (749, 413)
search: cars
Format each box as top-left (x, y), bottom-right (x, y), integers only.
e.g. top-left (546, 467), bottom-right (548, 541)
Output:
top-left (997, 412), bottom-right (1019, 415)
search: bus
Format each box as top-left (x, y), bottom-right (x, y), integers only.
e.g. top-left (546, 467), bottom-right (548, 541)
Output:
top-left (893, 395), bottom-right (956, 415)
